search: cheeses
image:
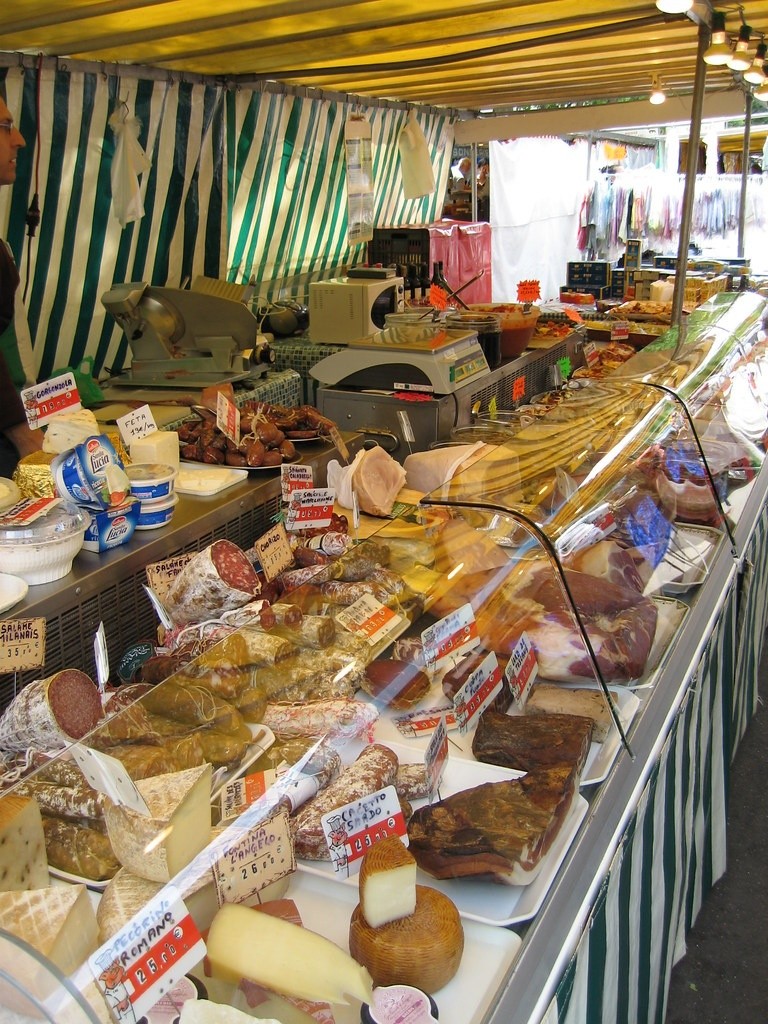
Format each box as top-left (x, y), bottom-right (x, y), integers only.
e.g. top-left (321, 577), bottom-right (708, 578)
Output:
top-left (0, 763), bottom-right (465, 1024)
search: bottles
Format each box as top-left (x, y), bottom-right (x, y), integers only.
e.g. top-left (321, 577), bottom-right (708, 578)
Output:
top-left (359, 261), bottom-right (447, 300)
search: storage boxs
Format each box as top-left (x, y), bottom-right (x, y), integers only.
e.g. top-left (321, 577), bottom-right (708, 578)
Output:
top-left (559, 239), bottom-right (751, 303)
top-left (368, 228), bottom-right (430, 279)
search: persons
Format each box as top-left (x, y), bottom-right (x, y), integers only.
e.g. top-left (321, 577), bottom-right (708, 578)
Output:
top-left (0, 92), bottom-right (46, 480)
top-left (444, 157), bottom-right (489, 223)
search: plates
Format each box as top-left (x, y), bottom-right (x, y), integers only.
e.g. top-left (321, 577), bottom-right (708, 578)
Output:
top-left (0, 570), bottom-right (28, 615)
top-left (180, 450), bottom-right (304, 470)
top-left (283, 436), bottom-right (334, 443)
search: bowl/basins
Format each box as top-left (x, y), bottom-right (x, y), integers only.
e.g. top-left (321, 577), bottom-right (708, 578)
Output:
top-left (459, 302), bottom-right (542, 357)
top-left (445, 312), bottom-right (502, 368)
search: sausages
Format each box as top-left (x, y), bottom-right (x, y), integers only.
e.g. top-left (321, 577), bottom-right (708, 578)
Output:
top-left (176, 402), bottom-right (337, 466)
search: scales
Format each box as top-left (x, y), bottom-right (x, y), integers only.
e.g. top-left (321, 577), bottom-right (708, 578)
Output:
top-left (308, 329), bottom-right (491, 395)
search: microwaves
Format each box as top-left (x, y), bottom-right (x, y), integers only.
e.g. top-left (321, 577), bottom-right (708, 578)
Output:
top-left (309, 277), bottom-right (404, 345)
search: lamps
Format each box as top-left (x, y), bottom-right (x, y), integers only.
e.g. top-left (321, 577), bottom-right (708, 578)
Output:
top-left (650, 72), bottom-right (666, 105)
top-left (753, 64), bottom-right (768, 102)
top-left (730, 31), bottom-right (767, 84)
top-left (703, 12), bottom-right (736, 65)
top-left (727, 8), bottom-right (752, 71)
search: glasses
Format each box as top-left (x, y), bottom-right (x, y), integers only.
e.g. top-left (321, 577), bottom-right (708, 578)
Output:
top-left (0, 122), bottom-right (15, 135)
